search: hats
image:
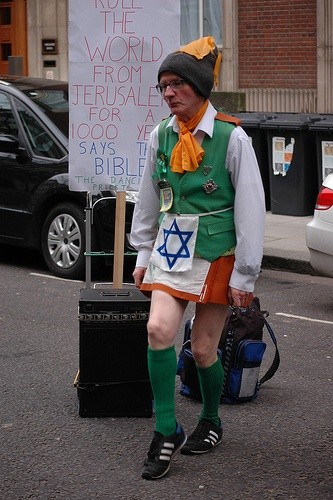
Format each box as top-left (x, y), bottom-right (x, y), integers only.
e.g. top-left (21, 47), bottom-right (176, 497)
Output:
top-left (158, 37), bottom-right (218, 98)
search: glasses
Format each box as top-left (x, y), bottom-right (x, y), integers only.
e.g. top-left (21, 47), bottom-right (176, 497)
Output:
top-left (156, 78), bottom-right (185, 94)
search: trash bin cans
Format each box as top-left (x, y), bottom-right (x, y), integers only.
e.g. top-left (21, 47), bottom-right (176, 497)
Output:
top-left (262, 112), bottom-right (325, 216)
top-left (226, 111), bottom-right (270, 212)
top-left (309, 113), bottom-right (333, 189)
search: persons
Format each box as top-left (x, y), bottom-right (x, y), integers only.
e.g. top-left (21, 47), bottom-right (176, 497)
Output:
top-left (129, 36), bottom-right (265, 480)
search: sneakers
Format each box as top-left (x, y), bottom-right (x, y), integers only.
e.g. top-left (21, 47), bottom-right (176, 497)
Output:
top-left (180, 416), bottom-right (223, 454)
top-left (141, 419), bottom-right (187, 479)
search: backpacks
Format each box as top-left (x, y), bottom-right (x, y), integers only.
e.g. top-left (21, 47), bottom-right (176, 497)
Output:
top-left (176, 297), bottom-right (280, 405)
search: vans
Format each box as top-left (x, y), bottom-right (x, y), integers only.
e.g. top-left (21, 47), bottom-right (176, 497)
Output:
top-left (1, 75), bottom-right (141, 278)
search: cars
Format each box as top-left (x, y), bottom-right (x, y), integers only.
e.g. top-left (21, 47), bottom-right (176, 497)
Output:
top-left (306, 172), bottom-right (333, 276)
